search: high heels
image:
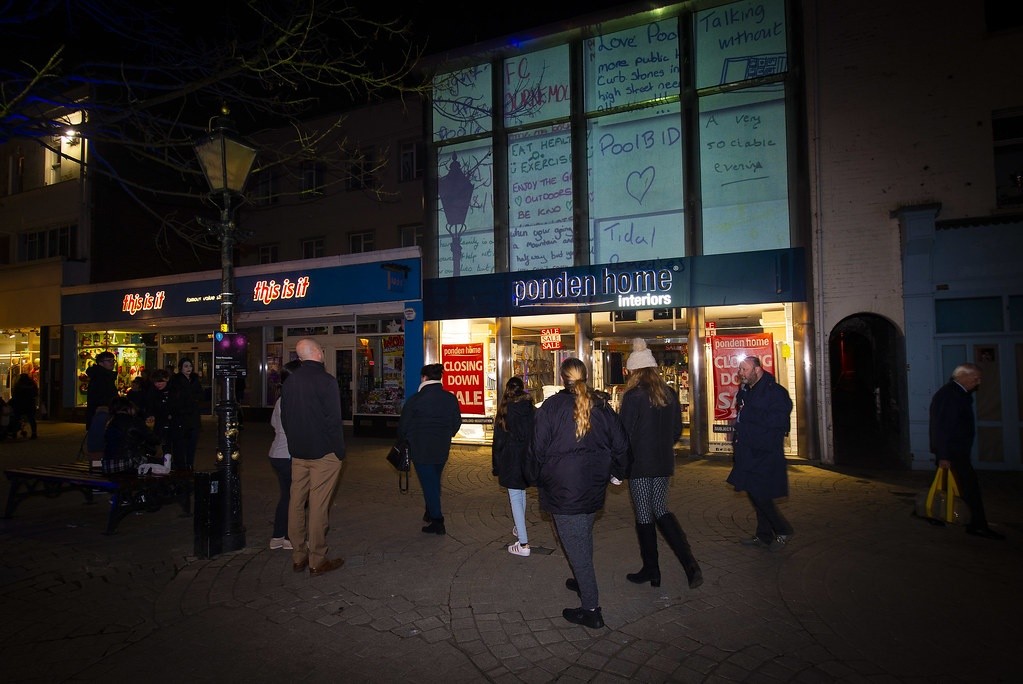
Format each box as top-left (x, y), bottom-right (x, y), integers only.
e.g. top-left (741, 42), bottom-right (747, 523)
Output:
top-left (422, 517), bottom-right (446, 535)
top-left (422, 510), bottom-right (432, 522)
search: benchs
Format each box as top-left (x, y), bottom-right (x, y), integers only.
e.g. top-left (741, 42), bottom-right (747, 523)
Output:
top-left (6, 458), bottom-right (198, 535)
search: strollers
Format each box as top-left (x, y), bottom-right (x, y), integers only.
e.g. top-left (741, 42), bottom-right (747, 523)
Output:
top-left (0, 398), bottom-right (27, 441)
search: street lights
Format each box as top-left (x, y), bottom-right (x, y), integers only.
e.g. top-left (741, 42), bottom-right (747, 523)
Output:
top-left (189, 105), bottom-right (259, 553)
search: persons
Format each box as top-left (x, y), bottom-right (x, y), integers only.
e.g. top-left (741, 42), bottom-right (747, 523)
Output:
top-left (11, 357), bottom-right (20, 376)
top-left (491, 377), bottom-right (537, 556)
top-left (268, 359), bottom-right (310, 549)
top-left (522, 358), bottom-right (630, 629)
top-left (30, 358), bottom-right (40, 384)
top-left (22, 354), bottom-right (32, 374)
top-left (9, 373), bottom-right (39, 439)
top-left (726, 357), bottom-right (794, 547)
top-left (618, 339), bottom-right (702, 590)
top-left (397, 363), bottom-right (462, 535)
top-left (280, 338), bottom-right (346, 577)
top-left (87, 352), bottom-right (206, 479)
top-left (924, 363), bottom-right (1006, 541)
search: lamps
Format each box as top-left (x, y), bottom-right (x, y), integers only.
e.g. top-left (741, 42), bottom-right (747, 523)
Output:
top-left (2, 328), bottom-right (40, 339)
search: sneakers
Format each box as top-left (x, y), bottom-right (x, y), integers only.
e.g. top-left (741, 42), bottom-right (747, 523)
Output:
top-left (507, 541), bottom-right (531, 556)
top-left (512, 526), bottom-right (518, 536)
top-left (566, 577), bottom-right (582, 597)
top-left (564, 604), bottom-right (604, 628)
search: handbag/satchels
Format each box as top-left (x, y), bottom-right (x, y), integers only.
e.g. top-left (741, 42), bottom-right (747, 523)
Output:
top-left (386, 439), bottom-right (410, 494)
top-left (138, 454), bottom-right (172, 475)
top-left (916, 465), bottom-right (969, 526)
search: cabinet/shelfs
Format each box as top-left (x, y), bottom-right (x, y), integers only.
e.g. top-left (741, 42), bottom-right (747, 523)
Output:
top-left (471, 323), bottom-right (554, 416)
top-left (357, 335), bottom-right (403, 415)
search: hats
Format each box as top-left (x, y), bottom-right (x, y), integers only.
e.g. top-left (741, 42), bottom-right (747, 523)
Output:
top-left (625, 338), bottom-right (658, 368)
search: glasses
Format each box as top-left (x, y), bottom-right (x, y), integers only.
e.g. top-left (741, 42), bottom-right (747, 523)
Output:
top-left (98, 358), bottom-right (116, 363)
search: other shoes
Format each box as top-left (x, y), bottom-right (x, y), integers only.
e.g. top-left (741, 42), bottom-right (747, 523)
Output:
top-left (283, 539), bottom-right (310, 549)
top-left (911, 509), bottom-right (946, 527)
top-left (966, 524), bottom-right (1006, 540)
top-left (739, 534), bottom-right (774, 547)
top-left (31, 433), bottom-right (37, 439)
top-left (769, 531), bottom-right (794, 552)
top-left (294, 559), bottom-right (307, 571)
top-left (309, 558), bottom-right (343, 575)
top-left (269, 537), bottom-right (285, 550)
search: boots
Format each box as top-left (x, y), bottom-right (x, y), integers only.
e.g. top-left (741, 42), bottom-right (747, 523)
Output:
top-left (627, 522), bottom-right (661, 587)
top-left (656, 512), bottom-right (704, 589)
